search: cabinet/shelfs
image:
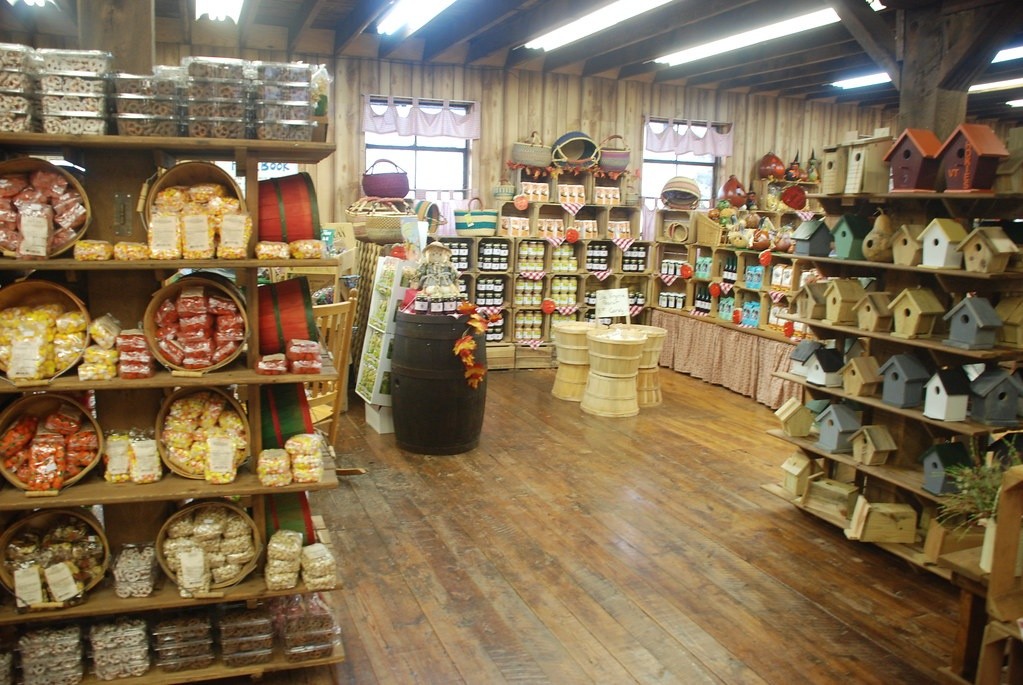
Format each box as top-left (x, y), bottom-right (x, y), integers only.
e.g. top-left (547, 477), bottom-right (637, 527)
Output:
top-left (753, 179), bottom-right (820, 209)
top-left (424, 163), bottom-right (651, 371)
top-left (652, 209), bottom-right (825, 412)
top-left (0, 132), bottom-right (345, 685)
top-left (759, 191), bottom-right (1023, 588)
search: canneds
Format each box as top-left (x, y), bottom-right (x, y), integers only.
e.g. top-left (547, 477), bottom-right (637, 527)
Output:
top-left (444, 243), bottom-right (687, 342)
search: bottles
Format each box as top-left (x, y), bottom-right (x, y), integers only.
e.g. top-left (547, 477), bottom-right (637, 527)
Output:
top-left (695, 285), bottom-right (711, 313)
top-left (719, 175), bottom-right (747, 208)
top-left (723, 257), bottom-right (737, 284)
top-left (758, 152), bottom-right (785, 180)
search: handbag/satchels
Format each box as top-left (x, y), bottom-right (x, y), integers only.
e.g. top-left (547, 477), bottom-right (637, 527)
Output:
top-left (551, 131), bottom-right (600, 170)
top-left (454, 198), bottom-right (498, 237)
top-left (668, 222), bottom-right (689, 242)
top-left (414, 200), bottom-right (448, 234)
top-left (661, 176), bottom-right (701, 210)
top-left (345, 159), bottom-right (417, 245)
top-left (598, 134), bottom-right (631, 172)
top-left (510, 131), bottom-right (553, 168)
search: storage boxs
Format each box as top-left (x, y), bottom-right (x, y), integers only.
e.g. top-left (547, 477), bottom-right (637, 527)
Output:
top-left (353, 257), bottom-right (418, 434)
top-left (0, 43), bottom-right (327, 142)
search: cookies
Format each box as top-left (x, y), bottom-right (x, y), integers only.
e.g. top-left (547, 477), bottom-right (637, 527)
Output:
top-left (0, 50), bottom-right (312, 141)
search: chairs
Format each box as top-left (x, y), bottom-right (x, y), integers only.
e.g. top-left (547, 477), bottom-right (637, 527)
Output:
top-left (304, 288), bottom-right (358, 448)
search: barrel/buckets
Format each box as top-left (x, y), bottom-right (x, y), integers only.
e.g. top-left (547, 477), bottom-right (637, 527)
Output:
top-left (391, 312), bottom-right (487, 454)
top-left (155, 498), bottom-right (263, 599)
top-left (1, 394), bottom-right (104, 497)
top-left (1, 156), bottom-right (90, 258)
top-left (258, 277), bottom-right (319, 354)
top-left (155, 387), bottom-right (251, 481)
top-left (264, 492), bottom-right (316, 546)
top-left (1, 281), bottom-right (90, 388)
top-left (258, 172), bottom-right (321, 246)
top-left (1, 508), bottom-right (109, 609)
top-left (259, 381), bottom-right (314, 450)
top-left (135, 160), bottom-right (244, 231)
top-left (142, 273), bottom-right (249, 377)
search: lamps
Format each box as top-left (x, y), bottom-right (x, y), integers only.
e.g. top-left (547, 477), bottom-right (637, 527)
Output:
top-left (376, 0), bottom-right (456, 38)
top-left (821, 45), bottom-right (1023, 90)
top-left (6, 0), bottom-right (61, 12)
top-left (195, 0), bottom-right (244, 25)
top-left (512, 0), bottom-right (673, 52)
top-left (1005, 99), bottom-right (1023, 108)
top-left (967, 78), bottom-right (1023, 94)
top-left (642, 0), bottom-right (887, 67)
